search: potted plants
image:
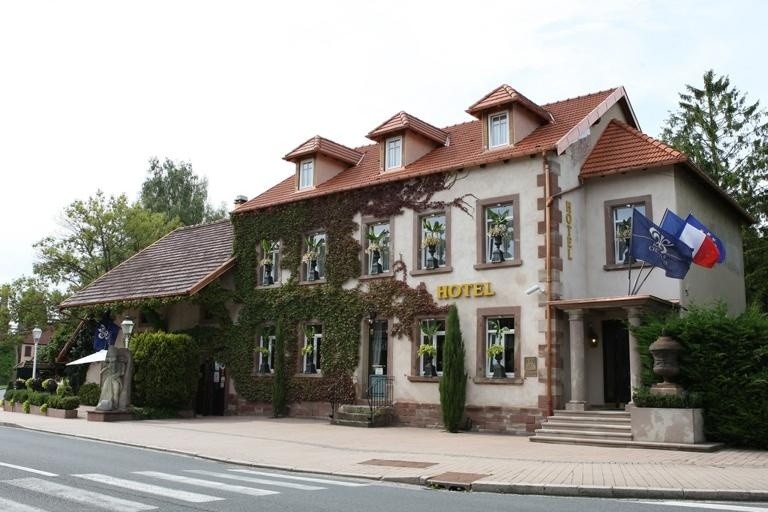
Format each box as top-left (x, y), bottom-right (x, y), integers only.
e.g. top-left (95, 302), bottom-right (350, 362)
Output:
top-left (247, 207), bottom-right (511, 286)
top-left (248, 317), bottom-right (511, 381)
top-left (618, 215), bottom-right (632, 264)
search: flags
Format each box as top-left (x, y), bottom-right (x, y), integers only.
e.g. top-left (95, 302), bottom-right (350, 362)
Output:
top-left (685, 213), bottom-right (725, 265)
top-left (629, 207), bottom-right (694, 280)
top-left (92, 321), bottom-right (120, 352)
top-left (659, 209), bottom-right (720, 269)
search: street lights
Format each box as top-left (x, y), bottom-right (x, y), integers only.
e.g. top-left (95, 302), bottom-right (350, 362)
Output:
top-left (29, 327), bottom-right (42, 378)
top-left (119, 319), bottom-right (136, 349)
